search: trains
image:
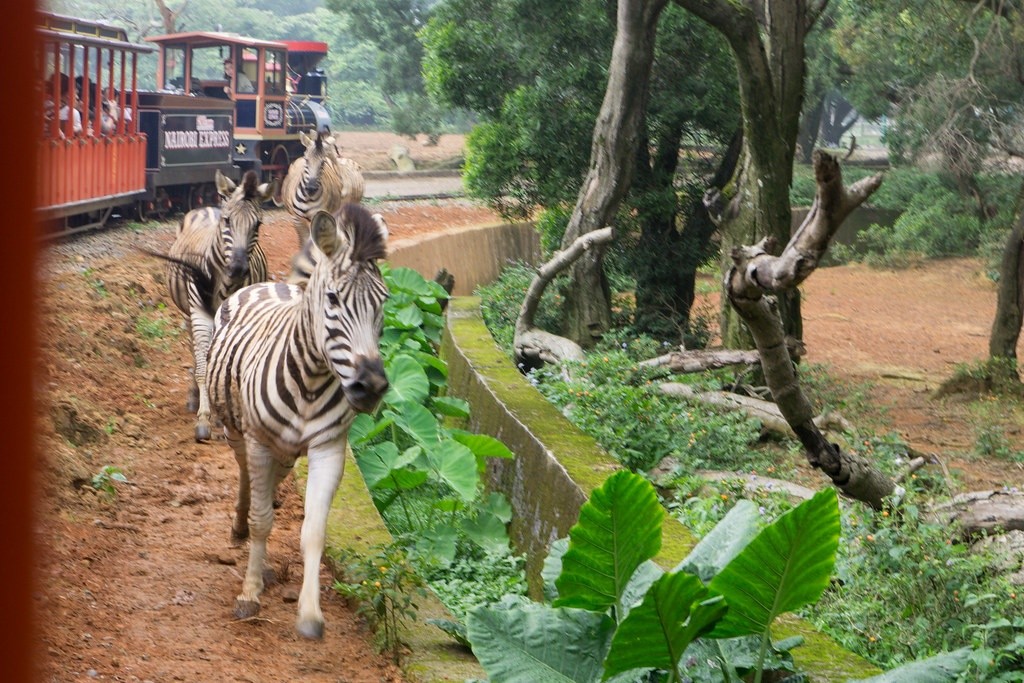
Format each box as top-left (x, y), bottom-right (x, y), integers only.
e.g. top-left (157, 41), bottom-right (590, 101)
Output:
top-left (34, 6), bottom-right (345, 241)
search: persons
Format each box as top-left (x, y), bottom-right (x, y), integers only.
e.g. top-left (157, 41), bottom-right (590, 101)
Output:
top-left (218, 58), bottom-right (255, 99)
top-left (38, 70), bottom-right (133, 141)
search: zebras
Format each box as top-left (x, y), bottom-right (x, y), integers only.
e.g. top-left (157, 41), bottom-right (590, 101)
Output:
top-left (136, 166), bottom-right (279, 444)
top-left (202, 204), bottom-right (389, 641)
top-left (280, 129), bottom-right (365, 253)
